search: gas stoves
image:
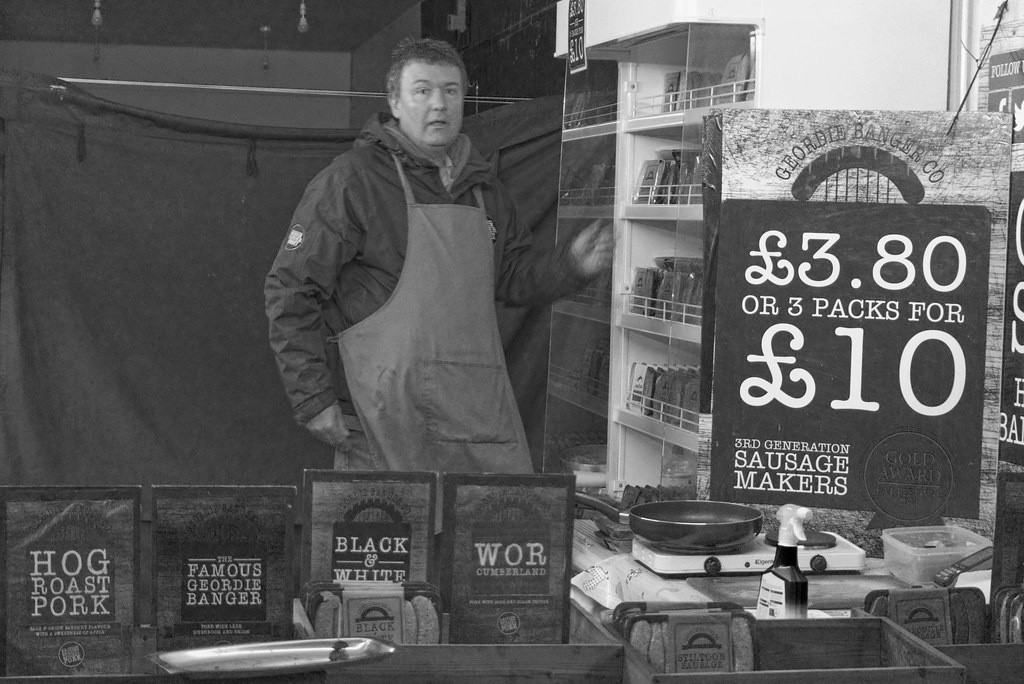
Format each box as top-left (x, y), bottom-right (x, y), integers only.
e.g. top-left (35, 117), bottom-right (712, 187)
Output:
top-left (629, 531), bottom-right (867, 575)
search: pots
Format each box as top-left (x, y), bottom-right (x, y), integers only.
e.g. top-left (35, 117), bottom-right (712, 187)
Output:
top-left (573, 490), bottom-right (762, 548)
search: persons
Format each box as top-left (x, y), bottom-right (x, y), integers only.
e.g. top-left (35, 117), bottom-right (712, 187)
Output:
top-left (261, 35), bottom-right (615, 592)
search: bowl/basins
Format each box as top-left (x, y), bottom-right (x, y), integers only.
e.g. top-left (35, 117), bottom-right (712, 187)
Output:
top-left (557, 444), bottom-right (608, 487)
